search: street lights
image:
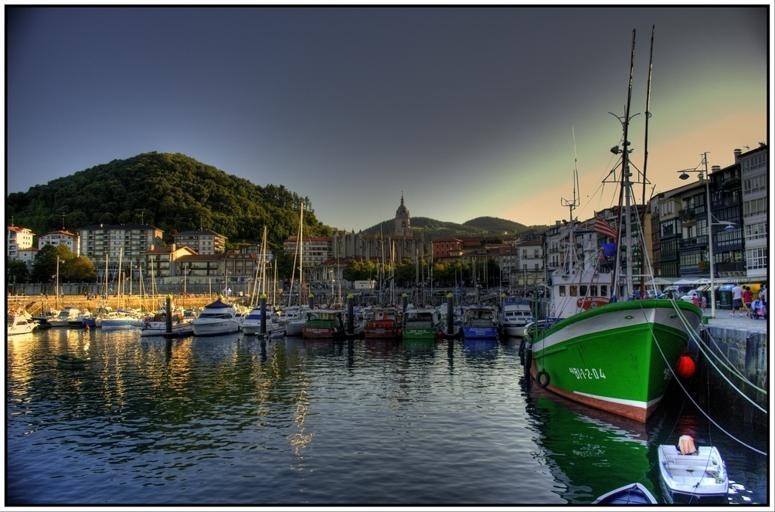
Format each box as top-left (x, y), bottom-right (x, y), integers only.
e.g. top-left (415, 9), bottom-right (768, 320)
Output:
top-left (675, 151), bottom-right (738, 316)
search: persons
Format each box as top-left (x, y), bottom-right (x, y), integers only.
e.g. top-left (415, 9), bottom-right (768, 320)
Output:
top-left (700, 294), bottom-right (707, 313)
top-left (731, 283), bottom-right (767, 320)
top-left (691, 293), bottom-right (699, 306)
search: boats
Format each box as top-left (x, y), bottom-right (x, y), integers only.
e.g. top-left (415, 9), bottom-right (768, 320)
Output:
top-left (657, 434), bottom-right (731, 504)
top-left (591, 482), bottom-right (659, 504)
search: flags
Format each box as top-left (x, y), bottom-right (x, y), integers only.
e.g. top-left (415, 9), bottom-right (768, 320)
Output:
top-left (593, 220), bottom-right (617, 238)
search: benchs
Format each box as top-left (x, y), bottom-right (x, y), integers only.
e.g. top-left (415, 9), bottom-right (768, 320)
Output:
top-left (666, 463), bottom-right (720, 475)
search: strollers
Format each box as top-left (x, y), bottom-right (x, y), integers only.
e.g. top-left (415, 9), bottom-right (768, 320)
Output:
top-left (750, 298), bottom-right (768, 320)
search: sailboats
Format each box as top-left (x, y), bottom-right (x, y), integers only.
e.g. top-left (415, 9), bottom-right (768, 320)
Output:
top-left (520, 22), bottom-right (702, 425)
